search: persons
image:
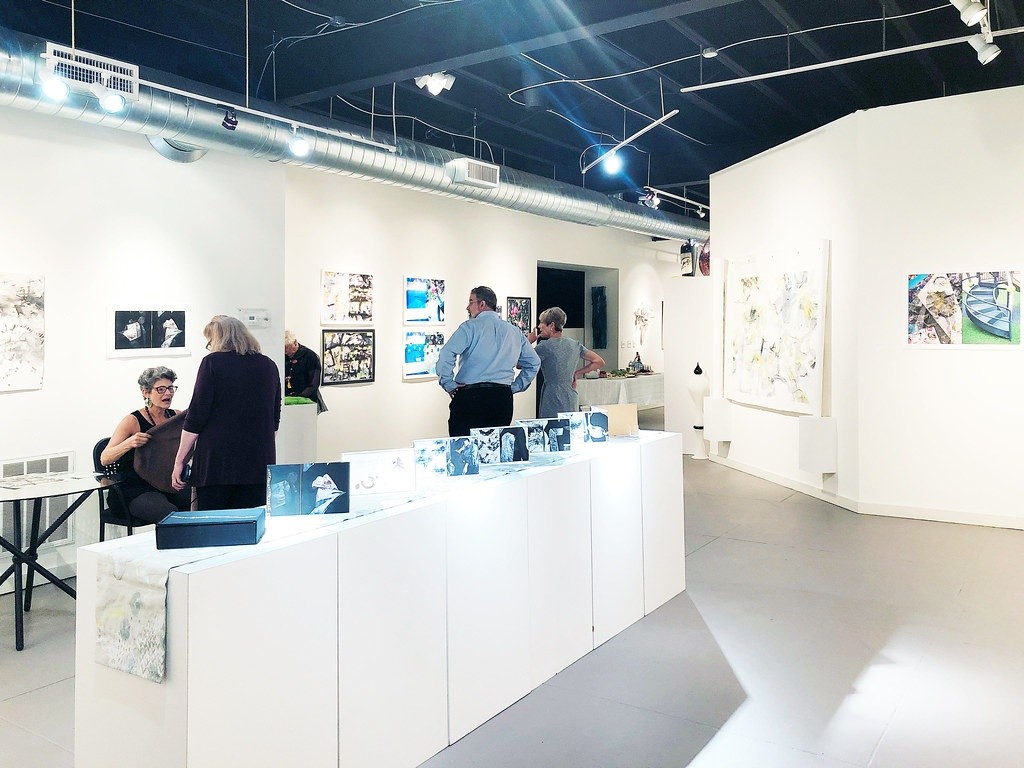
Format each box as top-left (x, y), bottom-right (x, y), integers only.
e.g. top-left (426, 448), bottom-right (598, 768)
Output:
top-left (434, 285), bottom-right (542, 438)
top-left (133, 314), bottom-right (282, 512)
top-left (526, 306), bottom-right (606, 421)
top-left (285, 330), bottom-right (328, 417)
top-left (99, 366), bottom-right (190, 523)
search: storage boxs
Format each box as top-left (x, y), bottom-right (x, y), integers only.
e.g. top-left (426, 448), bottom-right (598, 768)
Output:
top-left (155, 507), bottom-right (265, 551)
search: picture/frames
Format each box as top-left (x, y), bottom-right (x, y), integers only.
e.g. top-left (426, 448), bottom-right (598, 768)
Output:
top-left (400, 327), bottom-right (446, 380)
top-left (107, 303), bottom-right (193, 359)
top-left (403, 274), bottom-right (447, 327)
top-left (319, 269), bottom-right (375, 326)
top-left (320, 328), bottom-right (377, 388)
top-left (507, 296), bottom-right (532, 338)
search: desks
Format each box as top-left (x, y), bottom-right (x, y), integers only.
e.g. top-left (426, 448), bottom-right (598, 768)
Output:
top-left (0, 471), bottom-right (125, 652)
top-left (575, 373), bottom-right (664, 411)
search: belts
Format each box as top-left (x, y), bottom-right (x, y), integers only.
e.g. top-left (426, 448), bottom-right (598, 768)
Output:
top-left (458, 384), bottom-right (512, 390)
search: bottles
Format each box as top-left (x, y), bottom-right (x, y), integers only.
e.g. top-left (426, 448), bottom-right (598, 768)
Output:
top-left (694, 362), bottom-right (702, 374)
top-left (628, 351), bottom-right (644, 373)
top-left (680, 241), bottom-right (694, 276)
top-left (699, 237), bottom-right (710, 276)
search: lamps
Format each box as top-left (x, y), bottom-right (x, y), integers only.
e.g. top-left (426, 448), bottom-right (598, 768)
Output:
top-left (89, 73), bottom-right (127, 114)
top-left (218, 104), bottom-right (238, 132)
top-left (39, 59), bottom-right (70, 101)
top-left (968, 33), bottom-right (1002, 66)
top-left (288, 123), bottom-right (309, 158)
top-left (697, 206), bottom-right (705, 218)
top-left (950, 0), bottom-right (988, 27)
top-left (414, 71), bottom-right (457, 97)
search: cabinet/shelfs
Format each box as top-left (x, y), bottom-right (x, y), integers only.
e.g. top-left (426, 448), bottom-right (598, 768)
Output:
top-left (74, 429), bottom-right (686, 768)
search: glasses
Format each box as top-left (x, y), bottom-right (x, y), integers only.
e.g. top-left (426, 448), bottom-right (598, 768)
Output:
top-left (152, 385), bottom-right (178, 394)
top-left (205, 338), bottom-right (214, 352)
